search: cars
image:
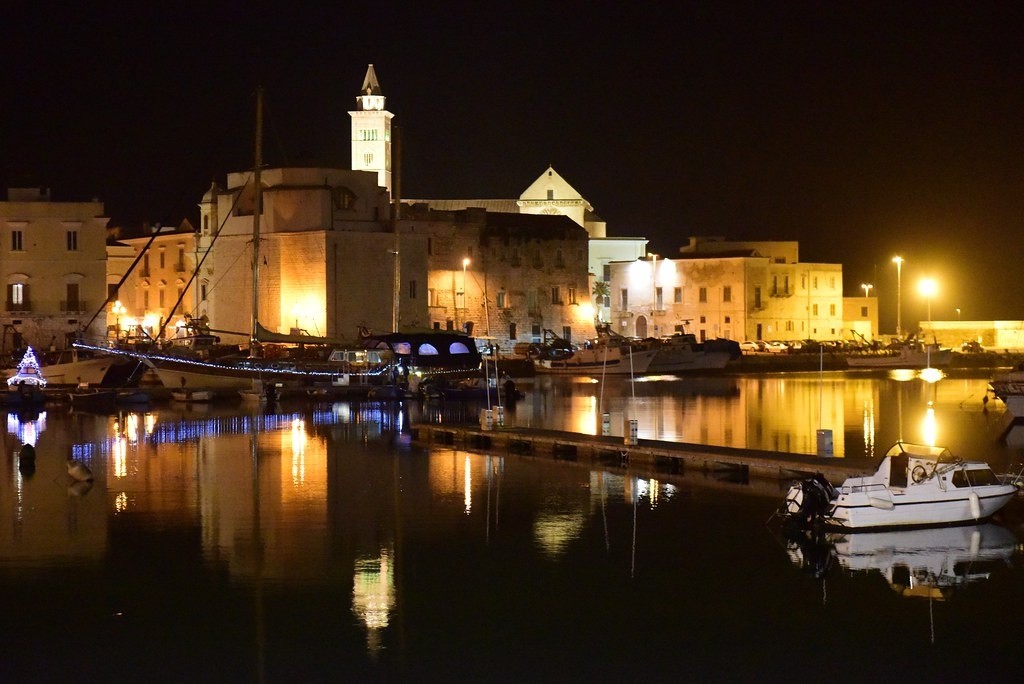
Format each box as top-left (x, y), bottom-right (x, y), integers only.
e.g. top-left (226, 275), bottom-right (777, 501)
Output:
top-left (741, 339), bottom-right (846, 355)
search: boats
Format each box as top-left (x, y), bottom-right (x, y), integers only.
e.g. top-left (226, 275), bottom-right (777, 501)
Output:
top-left (986, 358), bottom-right (1024, 417)
top-left (775, 440), bottom-right (1024, 534)
top-left (785, 521), bottom-right (1024, 643)
top-left (394, 363), bottom-right (526, 403)
top-left (632, 326), bottom-right (740, 375)
top-left (68, 352), bottom-right (173, 415)
top-left (171, 387), bottom-right (216, 403)
top-left (845, 329), bottom-right (936, 368)
top-left (113, 349), bottom-right (387, 400)
top-left (525, 316), bottom-right (659, 376)
top-left (0, 379), bottom-right (72, 427)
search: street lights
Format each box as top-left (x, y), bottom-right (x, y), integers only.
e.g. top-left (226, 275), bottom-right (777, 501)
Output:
top-left (462, 257), bottom-right (471, 323)
top-left (892, 255), bottom-right (904, 337)
top-left (860, 283), bottom-right (874, 297)
top-left (919, 280), bottom-right (934, 321)
top-left (112, 300), bottom-right (127, 340)
